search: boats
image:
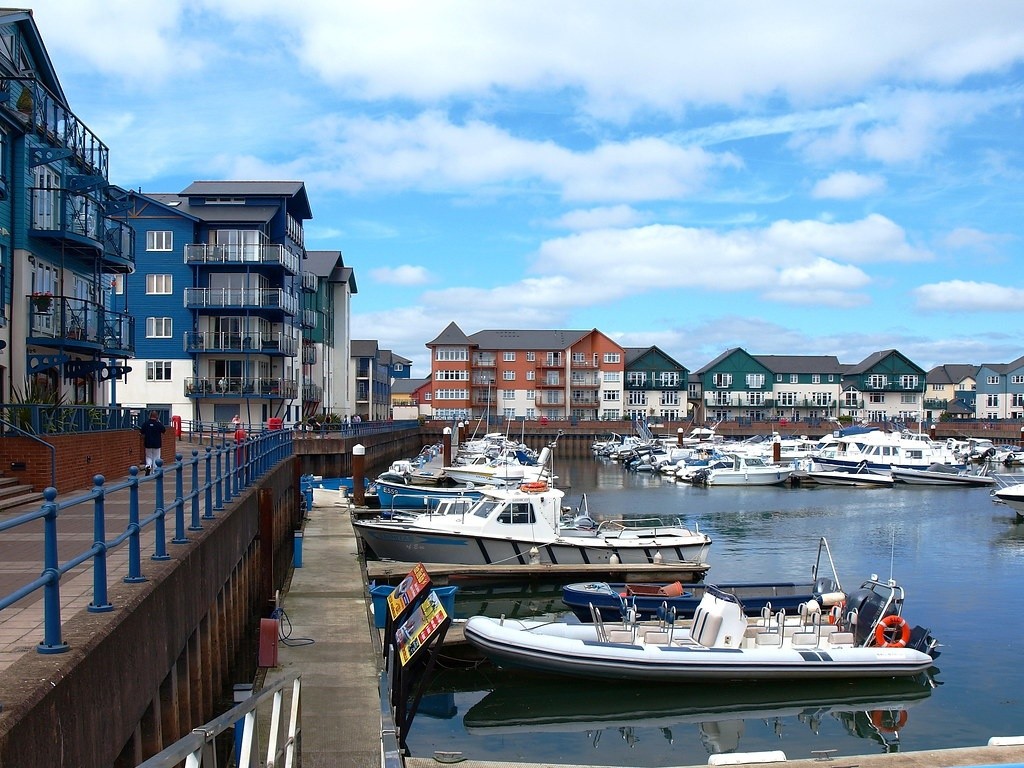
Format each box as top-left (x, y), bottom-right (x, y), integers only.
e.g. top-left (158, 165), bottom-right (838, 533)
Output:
top-left (989, 483), bottom-right (1024, 515)
top-left (366, 419), bottom-right (1023, 503)
top-left (350, 487), bottom-right (712, 580)
top-left (463, 572), bottom-right (938, 678)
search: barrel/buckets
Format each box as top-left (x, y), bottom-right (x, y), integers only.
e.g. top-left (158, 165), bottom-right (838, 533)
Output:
top-left (662, 581), bottom-right (684, 597)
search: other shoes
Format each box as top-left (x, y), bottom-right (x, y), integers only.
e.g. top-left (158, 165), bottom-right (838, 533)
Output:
top-left (295, 437), bottom-right (298, 439)
top-left (307, 437), bottom-right (311, 438)
top-left (145, 467), bottom-right (149, 476)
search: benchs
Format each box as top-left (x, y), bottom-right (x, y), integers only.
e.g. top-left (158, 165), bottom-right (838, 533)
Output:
top-left (671, 605), bottom-right (723, 649)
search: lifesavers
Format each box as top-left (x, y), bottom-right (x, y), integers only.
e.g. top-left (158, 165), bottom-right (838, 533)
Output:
top-left (525, 482), bottom-right (546, 488)
top-left (873, 710), bottom-right (908, 733)
top-left (876, 615), bottom-right (911, 648)
top-left (829, 600), bottom-right (846, 625)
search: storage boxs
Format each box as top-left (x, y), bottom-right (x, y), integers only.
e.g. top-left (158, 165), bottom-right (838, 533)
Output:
top-left (413, 586), bottom-right (459, 626)
top-left (368, 585), bottom-right (407, 629)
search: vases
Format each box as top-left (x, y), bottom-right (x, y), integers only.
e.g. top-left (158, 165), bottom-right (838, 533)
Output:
top-left (37, 305), bottom-right (47, 312)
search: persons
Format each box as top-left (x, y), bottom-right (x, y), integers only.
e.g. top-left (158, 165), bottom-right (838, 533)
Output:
top-left (293, 420), bottom-right (303, 439)
top-left (343, 414), bottom-right (351, 437)
top-left (353, 413), bottom-right (361, 435)
top-left (435, 471), bottom-right (459, 489)
top-left (306, 416), bottom-right (321, 439)
top-left (140, 410), bottom-right (166, 476)
top-left (232, 415), bottom-right (241, 431)
top-left (218, 378), bottom-right (228, 392)
top-left (324, 413), bottom-right (332, 434)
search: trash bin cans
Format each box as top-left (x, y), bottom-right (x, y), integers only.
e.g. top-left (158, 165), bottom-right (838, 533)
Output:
top-left (267, 417), bottom-right (282, 431)
top-left (172, 415), bottom-right (182, 437)
top-left (233, 428), bottom-right (245, 476)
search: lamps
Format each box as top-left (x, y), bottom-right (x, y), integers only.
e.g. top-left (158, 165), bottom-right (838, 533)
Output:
top-left (28, 255), bottom-right (35, 262)
top-left (107, 279), bottom-right (116, 290)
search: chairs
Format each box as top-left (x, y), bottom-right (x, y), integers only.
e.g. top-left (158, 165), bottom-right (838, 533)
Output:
top-left (605, 598), bottom-right (676, 647)
top-left (619, 726), bottom-right (674, 749)
top-left (762, 712), bottom-right (856, 739)
top-left (743, 601), bottom-right (858, 650)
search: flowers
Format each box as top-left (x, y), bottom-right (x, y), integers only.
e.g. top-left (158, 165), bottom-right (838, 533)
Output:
top-left (33, 291), bottom-right (53, 307)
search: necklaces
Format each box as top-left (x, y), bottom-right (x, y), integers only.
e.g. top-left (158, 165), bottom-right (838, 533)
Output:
top-left (440, 478), bottom-right (446, 481)
